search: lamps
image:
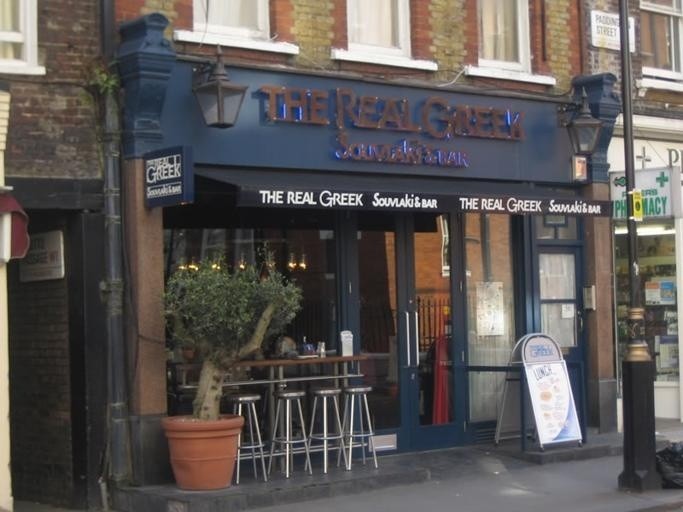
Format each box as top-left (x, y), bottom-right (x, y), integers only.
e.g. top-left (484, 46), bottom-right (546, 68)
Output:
top-left (193, 45), bottom-right (249, 128)
top-left (557, 85), bottom-right (602, 155)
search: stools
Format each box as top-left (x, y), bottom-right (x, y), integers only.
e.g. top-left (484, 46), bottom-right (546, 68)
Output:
top-left (233, 386), bottom-right (378, 485)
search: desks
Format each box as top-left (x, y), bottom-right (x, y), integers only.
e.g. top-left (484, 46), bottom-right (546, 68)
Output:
top-left (177, 353), bottom-right (390, 473)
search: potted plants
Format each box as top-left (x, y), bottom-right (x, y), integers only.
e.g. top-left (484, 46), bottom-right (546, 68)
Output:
top-left (155, 239), bottom-right (305, 491)
